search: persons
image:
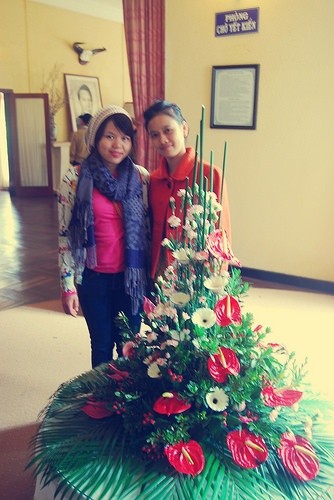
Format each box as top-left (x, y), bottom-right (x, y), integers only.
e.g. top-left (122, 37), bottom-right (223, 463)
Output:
top-left (75, 84), bottom-right (95, 130)
top-left (69, 113), bottom-right (94, 167)
top-left (143, 99), bottom-right (231, 336)
top-left (57, 104), bottom-right (151, 369)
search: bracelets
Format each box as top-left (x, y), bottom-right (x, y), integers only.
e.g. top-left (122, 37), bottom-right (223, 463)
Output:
top-left (62, 292), bottom-right (77, 296)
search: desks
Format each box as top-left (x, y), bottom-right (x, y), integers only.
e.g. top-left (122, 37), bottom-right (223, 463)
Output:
top-left (31, 355), bottom-right (333, 499)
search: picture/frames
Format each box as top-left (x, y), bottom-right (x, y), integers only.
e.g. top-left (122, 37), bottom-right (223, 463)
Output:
top-left (65, 73), bottom-right (103, 131)
top-left (210, 64), bottom-right (261, 129)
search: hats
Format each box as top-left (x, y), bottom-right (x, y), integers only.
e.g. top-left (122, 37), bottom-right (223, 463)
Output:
top-left (78, 113), bottom-right (94, 124)
top-left (85, 106), bottom-right (134, 155)
top-left (142, 99), bottom-right (173, 122)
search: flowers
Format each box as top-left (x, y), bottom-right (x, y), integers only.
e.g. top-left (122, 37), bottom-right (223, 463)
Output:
top-left (85, 106), bottom-right (322, 482)
